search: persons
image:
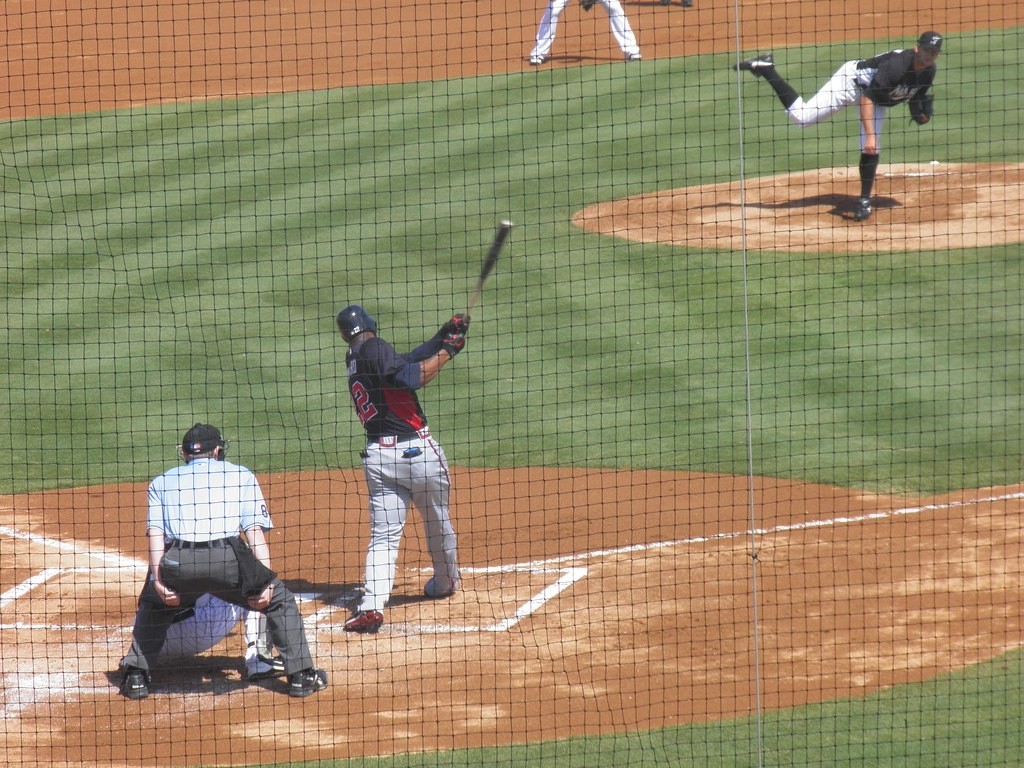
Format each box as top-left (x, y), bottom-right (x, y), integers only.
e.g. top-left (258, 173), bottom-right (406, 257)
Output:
top-left (529, 0), bottom-right (642, 65)
top-left (337, 305), bottom-right (471, 633)
top-left (136, 571), bottom-right (285, 680)
top-left (119, 422), bottom-right (328, 702)
top-left (733, 31), bottom-right (942, 218)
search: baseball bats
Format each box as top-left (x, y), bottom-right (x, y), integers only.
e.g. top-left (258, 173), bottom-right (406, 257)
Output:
top-left (462, 219), bottom-right (513, 324)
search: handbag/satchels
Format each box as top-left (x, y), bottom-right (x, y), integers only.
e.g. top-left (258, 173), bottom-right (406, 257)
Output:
top-left (229, 536), bottom-right (278, 598)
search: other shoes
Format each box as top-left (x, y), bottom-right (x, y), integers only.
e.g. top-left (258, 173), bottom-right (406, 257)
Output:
top-left (629, 54), bottom-right (642, 61)
top-left (424, 578), bottom-right (458, 597)
top-left (530, 55), bottom-right (542, 64)
top-left (343, 609), bottom-right (383, 632)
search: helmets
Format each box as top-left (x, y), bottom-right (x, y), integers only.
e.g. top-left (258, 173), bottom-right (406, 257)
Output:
top-left (336, 304), bottom-right (377, 343)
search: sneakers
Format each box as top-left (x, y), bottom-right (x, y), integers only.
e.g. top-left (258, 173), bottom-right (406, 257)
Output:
top-left (119, 669), bottom-right (149, 700)
top-left (733, 53), bottom-right (773, 77)
top-left (855, 197), bottom-right (872, 221)
top-left (248, 659), bottom-right (285, 680)
top-left (288, 669), bottom-right (328, 697)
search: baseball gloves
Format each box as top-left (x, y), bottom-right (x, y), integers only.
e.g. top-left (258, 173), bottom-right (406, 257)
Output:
top-left (910, 92), bottom-right (934, 125)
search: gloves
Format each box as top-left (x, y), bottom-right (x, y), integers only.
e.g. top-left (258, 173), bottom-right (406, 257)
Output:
top-left (440, 313), bottom-right (471, 360)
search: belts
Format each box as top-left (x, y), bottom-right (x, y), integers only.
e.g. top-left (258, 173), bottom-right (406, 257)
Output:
top-left (167, 538), bottom-right (229, 549)
top-left (367, 432), bottom-right (421, 444)
top-left (171, 609), bottom-right (195, 624)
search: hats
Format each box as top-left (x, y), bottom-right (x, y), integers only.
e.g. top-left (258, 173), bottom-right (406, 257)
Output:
top-left (919, 32), bottom-right (943, 52)
top-left (182, 422), bottom-right (221, 455)
